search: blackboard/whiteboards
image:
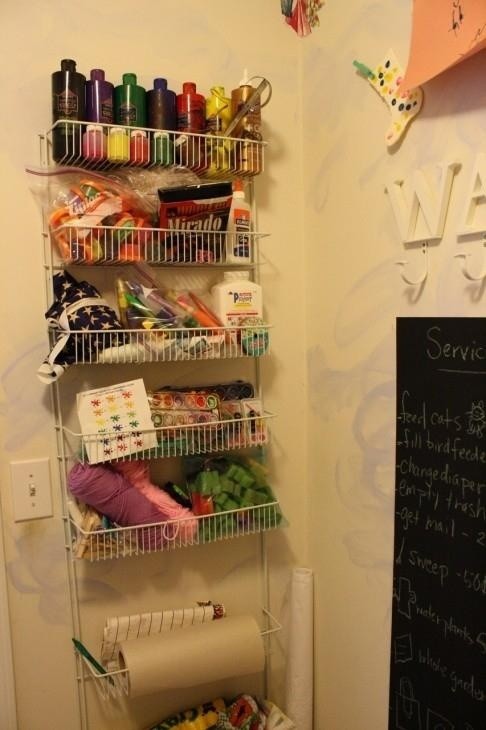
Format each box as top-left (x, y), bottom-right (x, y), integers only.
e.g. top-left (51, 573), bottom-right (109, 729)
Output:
top-left (388, 317), bottom-right (486, 730)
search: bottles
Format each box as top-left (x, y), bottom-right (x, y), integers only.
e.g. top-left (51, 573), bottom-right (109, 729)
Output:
top-left (50, 57), bottom-right (262, 176)
top-left (209, 271), bottom-right (262, 358)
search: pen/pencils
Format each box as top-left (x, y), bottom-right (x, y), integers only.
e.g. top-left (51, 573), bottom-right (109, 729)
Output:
top-left (72, 638), bottom-right (115, 686)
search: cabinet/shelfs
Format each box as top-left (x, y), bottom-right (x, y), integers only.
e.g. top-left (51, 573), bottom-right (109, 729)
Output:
top-left (36, 119), bottom-right (286, 730)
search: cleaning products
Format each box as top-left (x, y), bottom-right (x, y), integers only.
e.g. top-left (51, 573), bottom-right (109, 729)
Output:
top-left (232, 69), bottom-right (262, 174)
